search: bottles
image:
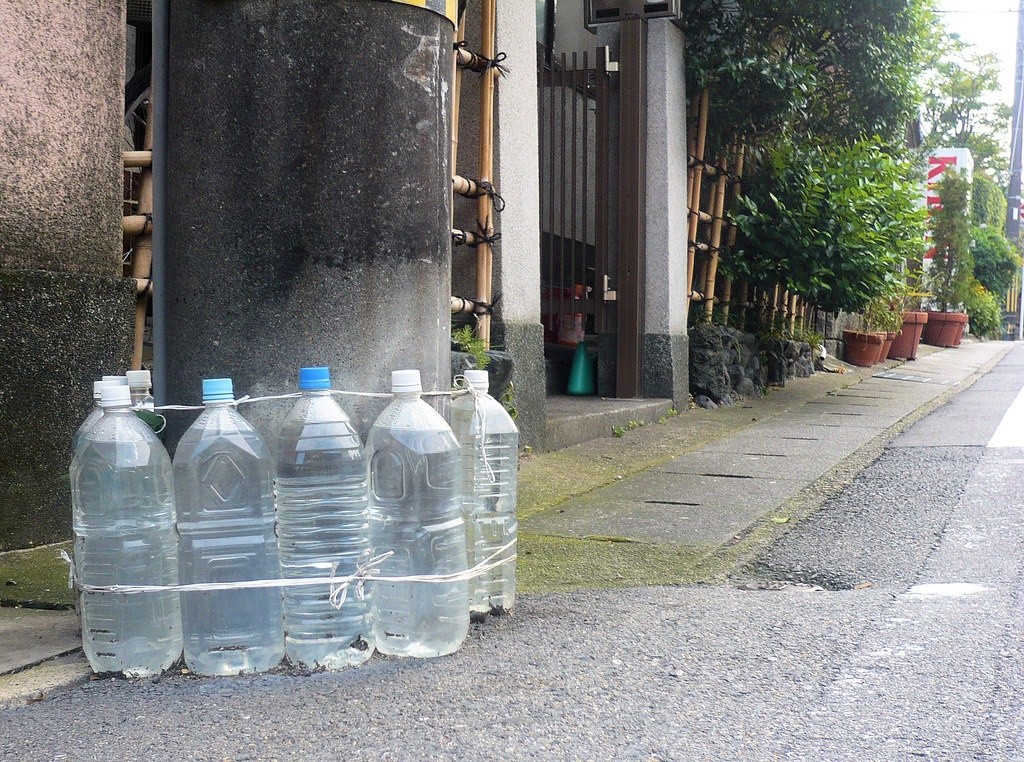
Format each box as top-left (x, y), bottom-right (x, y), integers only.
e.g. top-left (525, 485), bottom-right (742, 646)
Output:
top-left (71, 370), bottom-right (519, 678)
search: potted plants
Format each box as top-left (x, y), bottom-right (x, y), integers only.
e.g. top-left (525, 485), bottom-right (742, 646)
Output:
top-left (842, 167), bottom-right (978, 367)
